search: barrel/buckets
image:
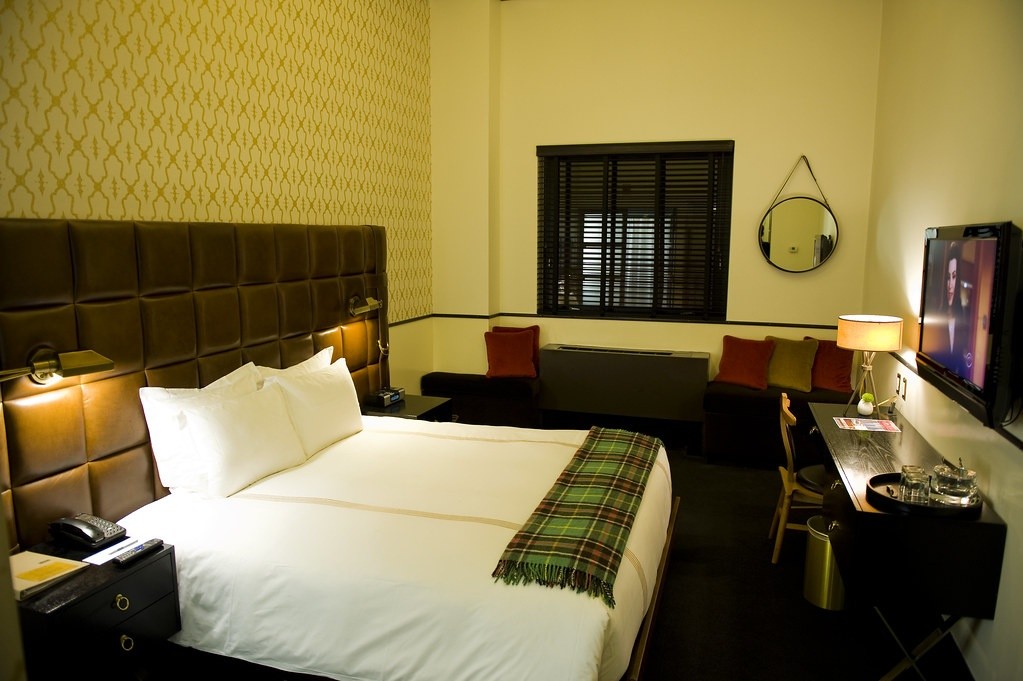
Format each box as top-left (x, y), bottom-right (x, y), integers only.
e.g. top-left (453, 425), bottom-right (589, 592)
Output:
top-left (805, 515), bottom-right (852, 611)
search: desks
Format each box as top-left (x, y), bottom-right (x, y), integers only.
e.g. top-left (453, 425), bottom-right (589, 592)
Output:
top-left (808, 402), bottom-right (1006, 681)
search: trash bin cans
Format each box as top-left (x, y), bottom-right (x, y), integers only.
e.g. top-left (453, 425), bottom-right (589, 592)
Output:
top-left (804, 515), bottom-right (845, 611)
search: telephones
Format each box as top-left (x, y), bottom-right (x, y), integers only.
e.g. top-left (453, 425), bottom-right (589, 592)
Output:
top-left (45, 512), bottom-right (127, 552)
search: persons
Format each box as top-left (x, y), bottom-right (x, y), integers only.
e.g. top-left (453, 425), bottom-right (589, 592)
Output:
top-left (932, 243), bottom-right (968, 380)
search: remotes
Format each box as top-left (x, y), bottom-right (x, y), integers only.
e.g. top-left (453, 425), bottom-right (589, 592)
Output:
top-left (112, 538), bottom-right (163, 569)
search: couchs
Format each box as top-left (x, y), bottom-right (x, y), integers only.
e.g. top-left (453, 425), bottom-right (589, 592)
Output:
top-left (418, 371), bottom-right (540, 428)
top-left (702, 382), bottom-right (860, 470)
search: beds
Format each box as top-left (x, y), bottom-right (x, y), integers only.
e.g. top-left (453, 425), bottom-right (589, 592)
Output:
top-left (1, 220), bottom-right (680, 681)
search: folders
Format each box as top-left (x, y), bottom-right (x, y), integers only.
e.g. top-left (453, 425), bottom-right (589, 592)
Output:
top-left (9, 549), bottom-right (91, 602)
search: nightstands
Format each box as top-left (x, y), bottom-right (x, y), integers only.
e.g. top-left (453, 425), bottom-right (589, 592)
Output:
top-left (361, 395), bottom-right (460, 423)
top-left (15, 535), bottom-right (182, 681)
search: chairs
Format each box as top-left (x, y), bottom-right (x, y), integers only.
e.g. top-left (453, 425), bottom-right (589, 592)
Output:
top-left (769, 393), bottom-right (826, 565)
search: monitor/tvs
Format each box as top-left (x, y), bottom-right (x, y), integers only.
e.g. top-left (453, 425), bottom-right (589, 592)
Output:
top-left (915, 222), bottom-right (1023, 429)
top-left (812, 235), bottom-right (832, 266)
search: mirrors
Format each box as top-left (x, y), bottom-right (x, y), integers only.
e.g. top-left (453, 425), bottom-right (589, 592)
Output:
top-left (758, 196), bottom-right (839, 273)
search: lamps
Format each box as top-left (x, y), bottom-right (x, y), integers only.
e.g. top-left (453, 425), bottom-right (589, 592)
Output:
top-left (836, 316), bottom-right (904, 419)
top-left (349, 296), bottom-right (386, 391)
top-left (0, 349), bottom-right (115, 384)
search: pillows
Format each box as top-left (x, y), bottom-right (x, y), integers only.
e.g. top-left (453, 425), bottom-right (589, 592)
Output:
top-left (804, 336), bottom-right (854, 393)
top-left (492, 324), bottom-right (540, 361)
top-left (764, 336), bottom-right (819, 392)
top-left (137, 346), bottom-right (364, 499)
top-left (484, 330), bottom-right (537, 378)
top-left (714, 335), bottom-right (776, 390)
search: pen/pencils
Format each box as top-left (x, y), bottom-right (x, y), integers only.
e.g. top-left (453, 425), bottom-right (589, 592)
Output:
top-left (110, 540), bottom-right (139, 555)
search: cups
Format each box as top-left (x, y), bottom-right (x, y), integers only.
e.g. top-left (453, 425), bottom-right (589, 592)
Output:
top-left (899, 465), bottom-right (930, 505)
